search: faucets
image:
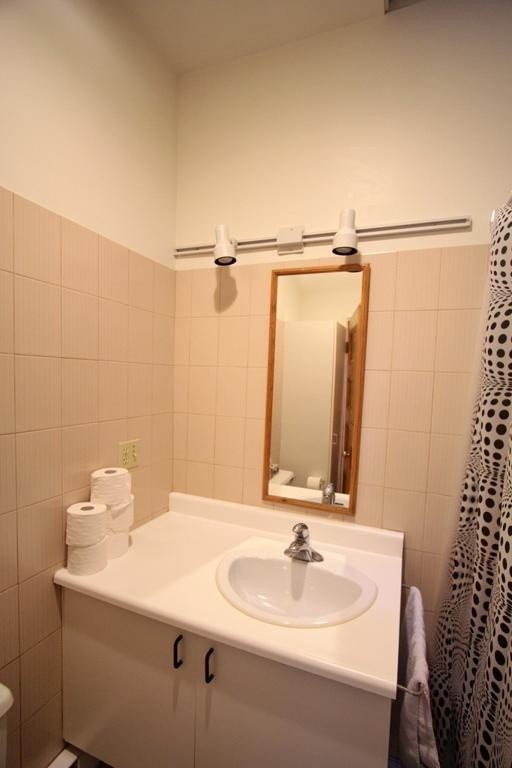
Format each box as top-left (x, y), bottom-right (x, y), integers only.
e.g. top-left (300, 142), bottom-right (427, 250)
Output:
top-left (321, 484), bottom-right (336, 504)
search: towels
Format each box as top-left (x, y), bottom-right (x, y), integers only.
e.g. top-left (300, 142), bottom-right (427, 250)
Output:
top-left (397, 585), bottom-right (440, 768)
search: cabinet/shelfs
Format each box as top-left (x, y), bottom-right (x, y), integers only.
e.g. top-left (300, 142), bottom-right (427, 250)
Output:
top-left (60, 586), bottom-right (393, 768)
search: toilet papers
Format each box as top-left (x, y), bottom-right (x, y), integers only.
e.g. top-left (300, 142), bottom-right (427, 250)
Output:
top-left (65, 467), bottom-right (135, 575)
top-left (305, 476), bottom-right (323, 490)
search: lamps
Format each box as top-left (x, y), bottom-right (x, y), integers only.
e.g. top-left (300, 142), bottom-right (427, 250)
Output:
top-left (172, 209), bottom-right (471, 266)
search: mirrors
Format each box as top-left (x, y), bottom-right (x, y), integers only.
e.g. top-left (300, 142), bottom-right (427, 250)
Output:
top-left (261, 264), bottom-right (371, 517)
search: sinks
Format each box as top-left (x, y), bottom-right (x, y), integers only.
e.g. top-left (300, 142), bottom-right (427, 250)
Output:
top-left (283, 522), bottom-right (323, 563)
top-left (213, 543), bottom-right (378, 629)
